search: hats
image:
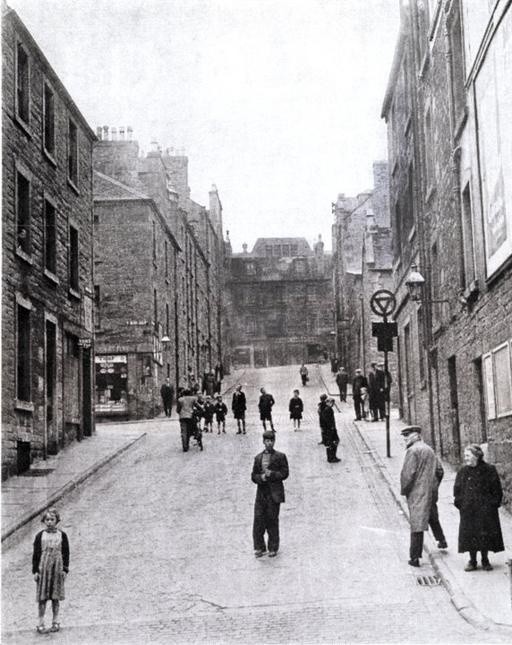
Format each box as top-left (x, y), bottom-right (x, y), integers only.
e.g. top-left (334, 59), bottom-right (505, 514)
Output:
top-left (401, 426), bottom-right (422, 434)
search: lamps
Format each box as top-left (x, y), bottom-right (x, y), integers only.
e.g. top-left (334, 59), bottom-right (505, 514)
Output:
top-left (153, 334), bottom-right (171, 354)
top-left (405, 265), bottom-right (446, 304)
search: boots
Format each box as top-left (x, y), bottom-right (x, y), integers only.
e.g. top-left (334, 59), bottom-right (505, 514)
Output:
top-left (326, 444), bottom-right (341, 463)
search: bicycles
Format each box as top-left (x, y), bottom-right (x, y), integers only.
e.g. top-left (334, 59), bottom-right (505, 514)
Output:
top-left (191, 408), bottom-right (204, 451)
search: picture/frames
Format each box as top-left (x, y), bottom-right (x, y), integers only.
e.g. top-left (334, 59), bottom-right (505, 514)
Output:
top-left (482, 353), bottom-right (496, 421)
top-left (492, 341), bottom-right (512, 418)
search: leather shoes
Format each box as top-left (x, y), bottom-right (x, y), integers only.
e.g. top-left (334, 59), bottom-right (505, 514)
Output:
top-left (50, 622), bottom-right (60, 632)
top-left (37, 625), bottom-right (49, 634)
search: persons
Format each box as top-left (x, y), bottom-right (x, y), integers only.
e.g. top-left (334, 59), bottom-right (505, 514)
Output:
top-left (258, 387), bottom-right (276, 432)
top-left (400, 427), bottom-right (447, 567)
top-left (161, 361), bottom-right (227, 451)
top-left (453, 444), bottom-right (502, 572)
top-left (289, 390), bottom-right (304, 432)
top-left (300, 363), bottom-right (308, 385)
top-left (32, 509), bottom-right (69, 634)
top-left (231, 385), bottom-right (246, 434)
top-left (251, 431), bottom-right (289, 559)
top-left (318, 362), bottom-right (386, 463)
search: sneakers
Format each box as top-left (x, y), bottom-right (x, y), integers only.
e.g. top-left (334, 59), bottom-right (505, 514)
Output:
top-left (408, 559), bottom-right (419, 566)
top-left (438, 542), bottom-right (447, 548)
top-left (269, 552), bottom-right (277, 556)
top-left (255, 550), bottom-right (264, 556)
top-left (482, 560), bottom-right (493, 570)
top-left (464, 560), bottom-right (478, 571)
